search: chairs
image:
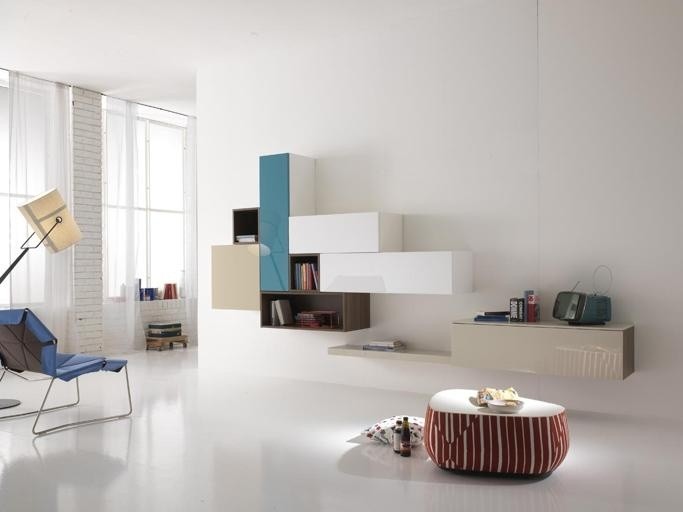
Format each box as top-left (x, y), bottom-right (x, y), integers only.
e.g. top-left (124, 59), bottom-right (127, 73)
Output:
top-left (0, 308), bottom-right (131, 436)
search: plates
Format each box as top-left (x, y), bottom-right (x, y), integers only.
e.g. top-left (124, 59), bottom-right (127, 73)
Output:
top-left (487, 399), bottom-right (524, 413)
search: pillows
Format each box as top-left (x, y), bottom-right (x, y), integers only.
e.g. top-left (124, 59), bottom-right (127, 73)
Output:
top-left (364, 416), bottom-right (424, 451)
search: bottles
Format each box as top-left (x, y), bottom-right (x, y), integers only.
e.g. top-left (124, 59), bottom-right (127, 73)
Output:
top-left (394, 417), bottom-right (411, 455)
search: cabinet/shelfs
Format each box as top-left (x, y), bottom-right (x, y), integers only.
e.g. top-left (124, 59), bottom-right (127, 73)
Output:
top-left (231, 208), bottom-right (368, 331)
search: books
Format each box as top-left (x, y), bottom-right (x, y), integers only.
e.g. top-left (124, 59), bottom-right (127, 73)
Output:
top-left (270, 262), bottom-right (322, 328)
top-left (369, 340), bottom-right (400, 346)
top-left (362, 345), bottom-right (406, 351)
top-left (474, 290), bottom-right (541, 322)
top-left (236, 235), bottom-right (258, 242)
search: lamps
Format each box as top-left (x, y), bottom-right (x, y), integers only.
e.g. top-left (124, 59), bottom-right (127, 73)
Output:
top-left (0, 189), bottom-right (82, 289)
